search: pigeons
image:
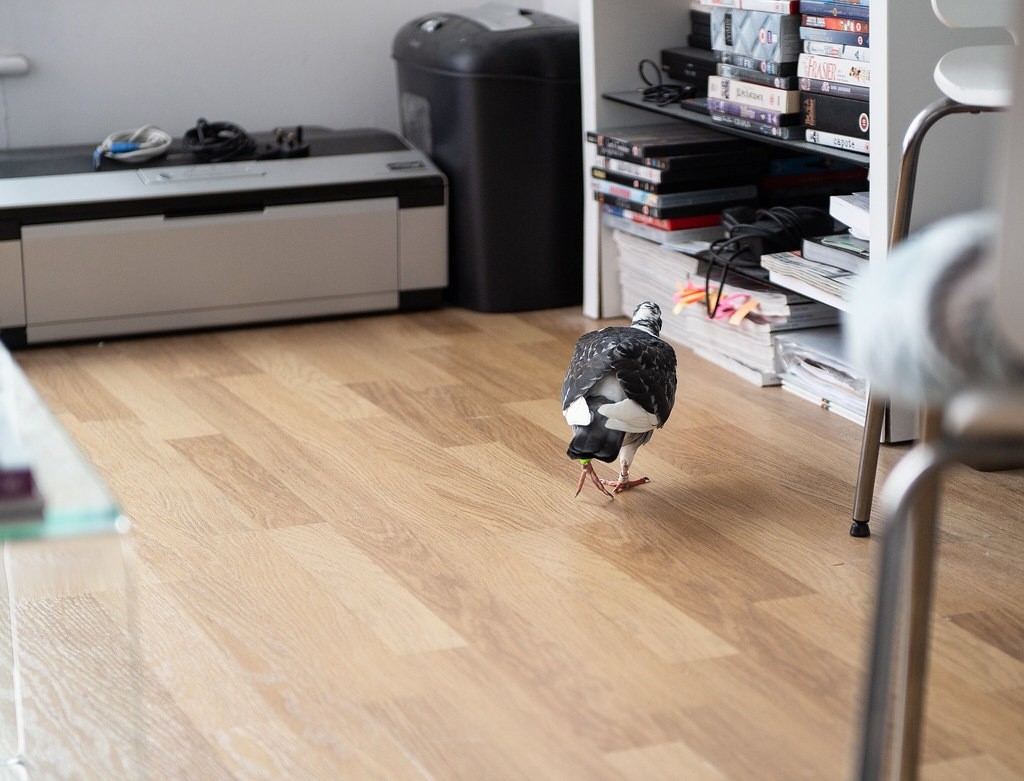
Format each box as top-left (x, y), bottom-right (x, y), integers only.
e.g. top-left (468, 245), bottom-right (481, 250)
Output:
top-left (557, 301), bottom-right (677, 499)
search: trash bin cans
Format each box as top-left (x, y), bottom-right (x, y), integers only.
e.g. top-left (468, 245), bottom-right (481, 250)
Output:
top-left (392, 5), bottom-right (584, 314)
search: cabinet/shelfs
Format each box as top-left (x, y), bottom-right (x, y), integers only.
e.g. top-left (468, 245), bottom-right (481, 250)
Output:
top-left (0, 124), bottom-right (452, 350)
top-left (576, 0), bottom-right (1015, 450)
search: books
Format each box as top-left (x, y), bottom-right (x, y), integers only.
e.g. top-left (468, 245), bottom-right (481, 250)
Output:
top-left (587, 0), bottom-right (874, 427)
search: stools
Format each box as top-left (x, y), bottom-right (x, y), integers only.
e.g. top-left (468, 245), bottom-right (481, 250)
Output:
top-left (832, 133), bottom-right (1024, 781)
top-left (848, 0), bottom-right (1024, 547)
